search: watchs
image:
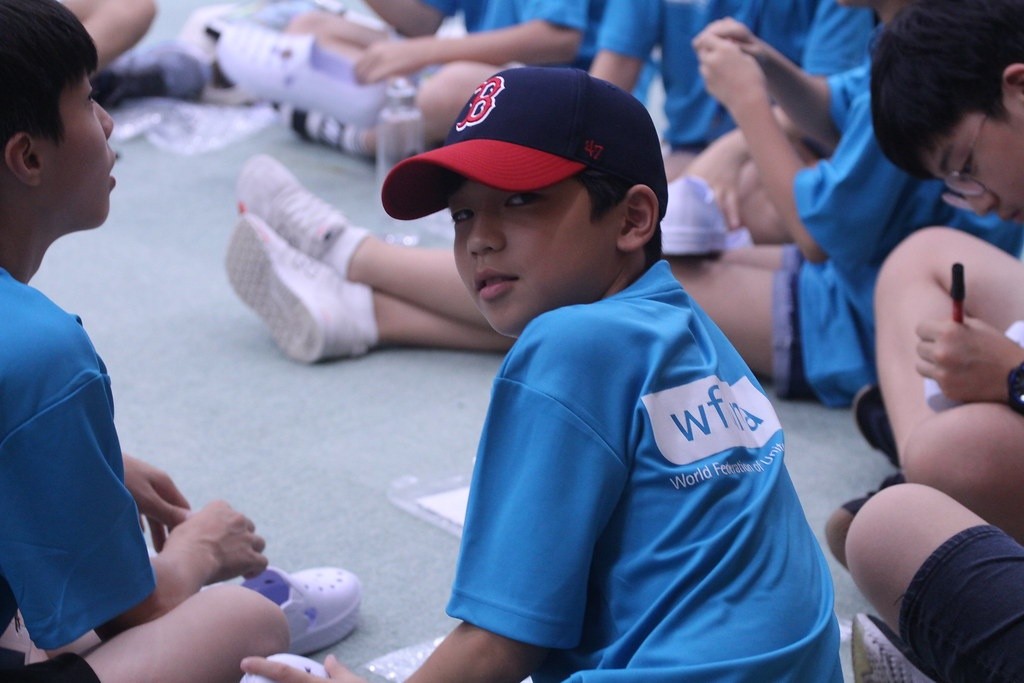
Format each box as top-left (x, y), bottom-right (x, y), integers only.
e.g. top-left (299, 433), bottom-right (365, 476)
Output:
top-left (1007, 362), bottom-right (1024, 417)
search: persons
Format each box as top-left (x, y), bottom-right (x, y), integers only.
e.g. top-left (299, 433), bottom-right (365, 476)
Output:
top-left (53, 0), bottom-right (1024, 683)
top-left (0, 0), bottom-right (360, 683)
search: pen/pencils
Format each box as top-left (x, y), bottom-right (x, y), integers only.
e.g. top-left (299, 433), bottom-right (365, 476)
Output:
top-left (948, 262), bottom-right (966, 320)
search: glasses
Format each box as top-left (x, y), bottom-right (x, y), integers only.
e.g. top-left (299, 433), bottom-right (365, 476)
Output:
top-left (942, 96), bottom-right (996, 213)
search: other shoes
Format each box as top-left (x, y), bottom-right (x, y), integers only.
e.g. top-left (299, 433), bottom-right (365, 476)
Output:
top-left (238, 652), bottom-right (327, 683)
top-left (854, 386), bottom-right (901, 470)
top-left (239, 566), bottom-right (363, 657)
top-left (851, 611), bottom-right (940, 683)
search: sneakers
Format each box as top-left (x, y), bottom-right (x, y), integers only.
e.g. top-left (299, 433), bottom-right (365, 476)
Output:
top-left (217, 24), bottom-right (386, 129)
top-left (281, 102), bottom-right (360, 155)
top-left (236, 153), bottom-right (350, 260)
top-left (659, 175), bottom-right (727, 254)
top-left (107, 47), bottom-right (209, 100)
top-left (226, 213), bottom-right (369, 364)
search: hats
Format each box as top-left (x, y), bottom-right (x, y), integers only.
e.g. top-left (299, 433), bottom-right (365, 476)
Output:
top-left (382, 68), bottom-right (668, 222)
